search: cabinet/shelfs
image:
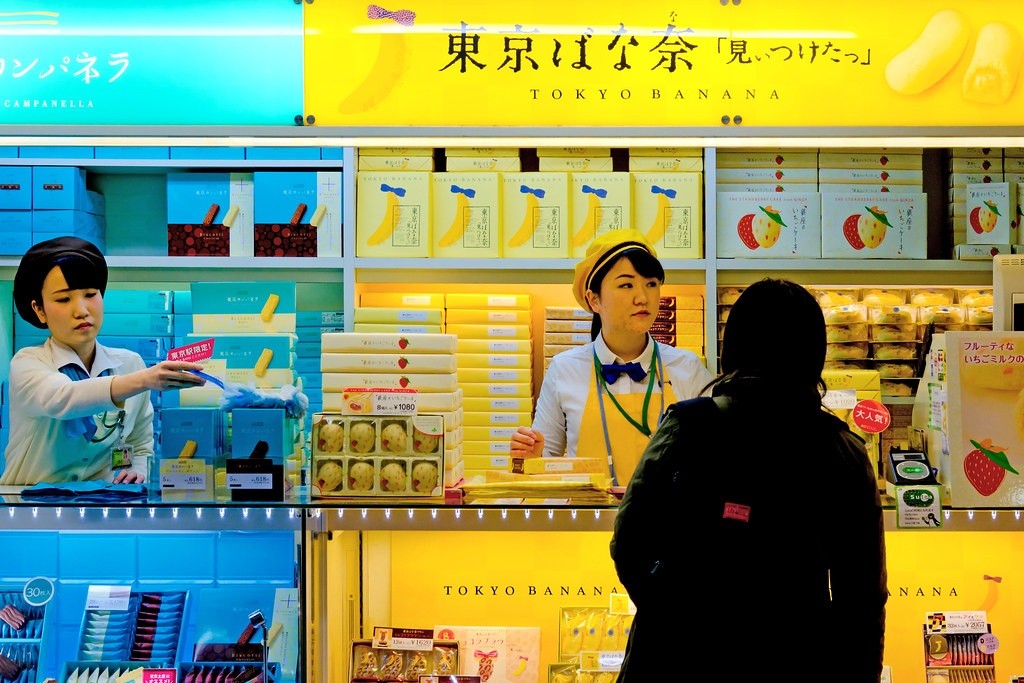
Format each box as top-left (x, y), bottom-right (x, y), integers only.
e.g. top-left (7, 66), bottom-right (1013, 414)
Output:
top-left (0, 138), bottom-right (1024, 377)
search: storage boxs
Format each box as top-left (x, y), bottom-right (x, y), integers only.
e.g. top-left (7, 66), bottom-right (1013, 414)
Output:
top-left (87, 189), bottom-right (97, 215)
top-left (96, 216), bottom-right (105, 237)
top-left (0, 146), bottom-right (94, 159)
top-left (0, 232), bottom-right (33, 255)
top-left (161, 147), bottom-right (1024, 506)
top-left (97, 193), bottom-right (105, 215)
top-left (15, 336), bottom-right (172, 360)
top-left (80, 169), bottom-right (87, 212)
top-left (33, 233), bottom-right (86, 247)
top-left (33, 210), bottom-right (86, 233)
top-left (0, 166), bottom-right (33, 210)
top-left (13, 290), bottom-right (172, 314)
top-left (33, 166), bottom-right (80, 210)
top-left (0, 210), bottom-right (33, 232)
top-left (95, 146), bottom-right (170, 159)
top-left (86, 213), bottom-right (96, 236)
top-left (86, 235), bottom-right (96, 244)
top-left (97, 238), bottom-right (106, 256)
top-left (0, 584), bottom-right (997, 683)
top-left (14, 313), bottom-right (174, 337)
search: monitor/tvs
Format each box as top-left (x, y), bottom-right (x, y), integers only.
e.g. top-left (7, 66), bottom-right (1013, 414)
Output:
top-left (992, 254), bottom-right (1024, 332)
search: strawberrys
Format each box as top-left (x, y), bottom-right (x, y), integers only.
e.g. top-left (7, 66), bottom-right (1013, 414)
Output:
top-left (982, 148), bottom-right (991, 183)
top-left (737, 214), bottom-right (763, 250)
top-left (843, 214), bottom-right (867, 250)
top-left (969, 207), bottom-right (985, 234)
top-left (880, 156), bottom-right (890, 192)
top-left (775, 155), bottom-right (784, 192)
top-left (398, 336), bottom-right (411, 388)
top-left (990, 247), bottom-right (999, 257)
top-left (964, 438), bottom-right (1020, 496)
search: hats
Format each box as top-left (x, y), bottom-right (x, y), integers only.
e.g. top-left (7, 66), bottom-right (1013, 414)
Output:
top-left (573, 228), bottom-right (657, 314)
top-left (14, 236), bottom-right (108, 329)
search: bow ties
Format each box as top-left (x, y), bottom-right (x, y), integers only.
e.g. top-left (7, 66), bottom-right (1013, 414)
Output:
top-left (601, 361), bottom-right (647, 385)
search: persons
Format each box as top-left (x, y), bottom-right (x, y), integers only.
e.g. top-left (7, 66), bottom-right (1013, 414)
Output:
top-left (509, 230), bottom-right (715, 493)
top-left (609, 279), bottom-right (887, 683)
top-left (121, 449), bottom-right (130, 465)
top-left (0, 236), bottom-right (207, 485)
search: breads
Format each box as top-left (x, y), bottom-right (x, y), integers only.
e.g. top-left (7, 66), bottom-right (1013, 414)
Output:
top-left (315, 423), bottom-right (438, 492)
top-left (815, 291), bottom-right (995, 396)
top-left (355, 649), bottom-right (455, 680)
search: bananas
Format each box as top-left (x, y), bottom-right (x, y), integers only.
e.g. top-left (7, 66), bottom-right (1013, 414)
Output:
top-left (365, 191), bottom-right (401, 245)
top-left (574, 193), bottom-right (602, 247)
top-left (508, 193), bottom-right (540, 248)
top-left (645, 193), bottom-right (671, 246)
top-left (438, 193), bottom-right (470, 247)
